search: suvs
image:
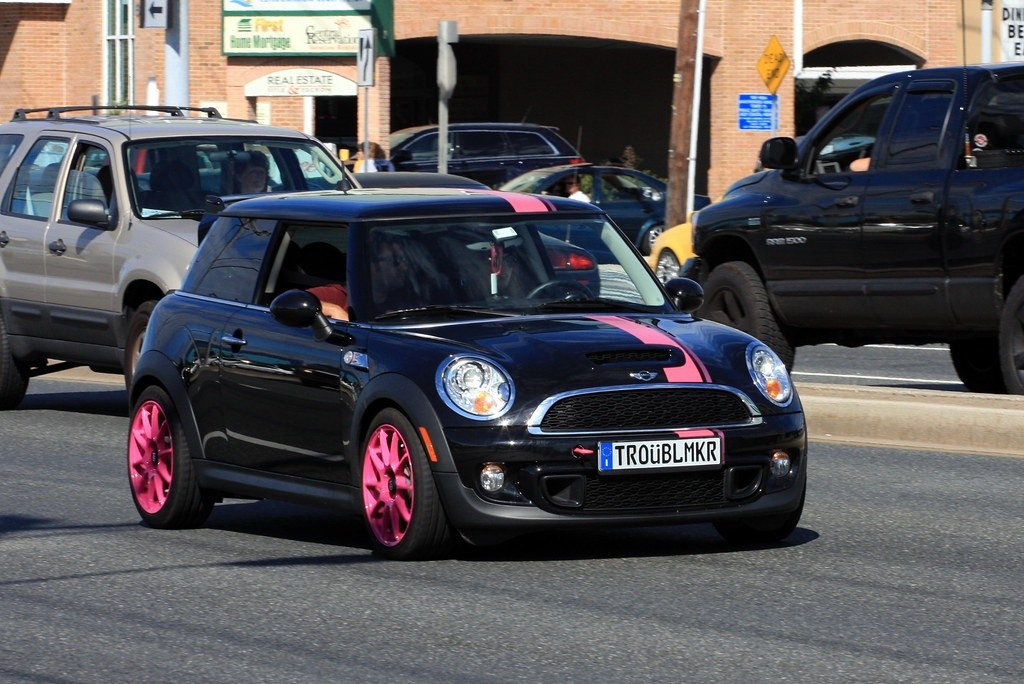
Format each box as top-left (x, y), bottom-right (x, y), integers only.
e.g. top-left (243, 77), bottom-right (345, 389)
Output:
top-left (0, 106), bottom-right (364, 412)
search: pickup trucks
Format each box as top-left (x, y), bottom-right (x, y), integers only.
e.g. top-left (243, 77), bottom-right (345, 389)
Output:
top-left (689, 63), bottom-right (1024, 396)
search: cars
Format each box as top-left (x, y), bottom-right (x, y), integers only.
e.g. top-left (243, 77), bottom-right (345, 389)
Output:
top-left (126, 190), bottom-right (809, 562)
top-left (648, 197), bottom-right (723, 288)
top-left (343, 121), bottom-right (584, 199)
top-left (498, 163), bottom-right (712, 258)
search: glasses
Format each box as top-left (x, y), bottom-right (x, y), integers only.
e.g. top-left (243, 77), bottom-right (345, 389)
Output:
top-left (487, 256), bottom-right (516, 269)
top-left (565, 181), bottom-right (579, 185)
top-left (376, 255), bottom-right (410, 266)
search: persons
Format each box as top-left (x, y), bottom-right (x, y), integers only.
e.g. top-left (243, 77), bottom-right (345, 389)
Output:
top-left (304, 231), bottom-right (410, 322)
top-left (354, 141), bottom-right (394, 173)
top-left (234, 149), bottom-right (270, 192)
top-left (564, 175), bottom-right (591, 203)
top-left (466, 244), bottom-right (544, 304)
